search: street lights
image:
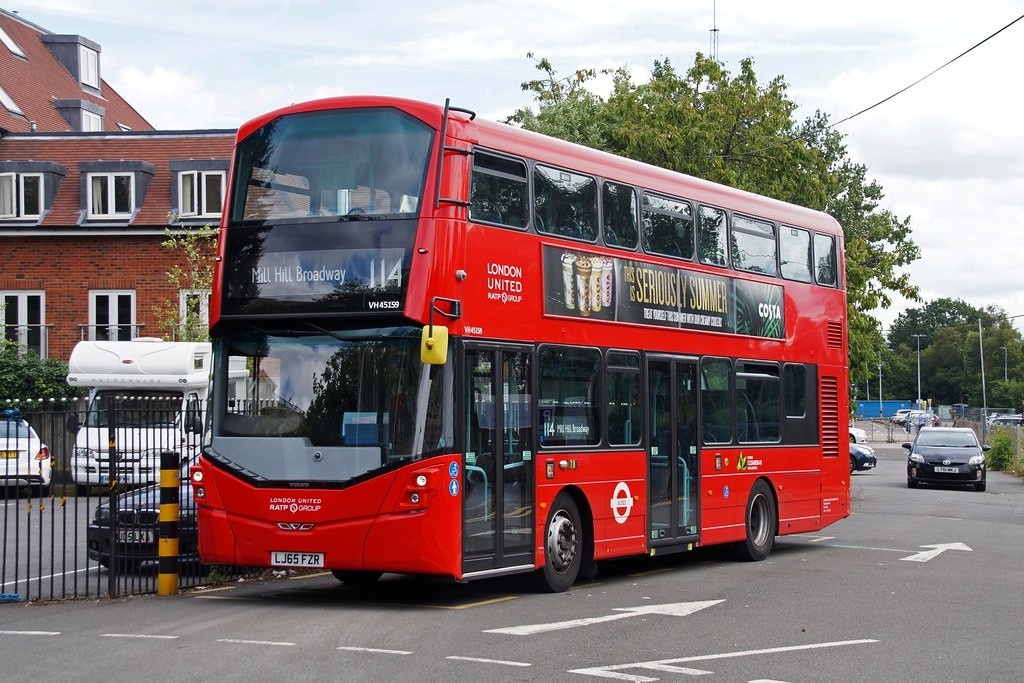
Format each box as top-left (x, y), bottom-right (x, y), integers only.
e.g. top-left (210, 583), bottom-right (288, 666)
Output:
top-left (999, 347), bottom-right (1007, 380)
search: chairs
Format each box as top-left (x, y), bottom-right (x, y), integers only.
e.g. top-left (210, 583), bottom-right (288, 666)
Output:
top-left (345, 202), bottom-right (833, 285)
top-left (718, 405), bottom-right (748, 442)
top-left (608, 416), bottom-right (632, 443)
top-left (755, 399), bottom-right (784, 441)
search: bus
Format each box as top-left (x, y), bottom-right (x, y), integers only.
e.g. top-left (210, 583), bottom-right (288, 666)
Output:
top-left (188, 95), bottom-right (851, 593)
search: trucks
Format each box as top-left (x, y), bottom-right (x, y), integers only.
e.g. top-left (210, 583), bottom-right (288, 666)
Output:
top-left (66, 337), bottom-right (250, 499)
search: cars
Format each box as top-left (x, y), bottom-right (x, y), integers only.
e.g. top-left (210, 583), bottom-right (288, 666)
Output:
top-left (890, 409), bottom-right (940, 434)
top-left (87, 451), bottom-right (253, 578)
top-left (849, 442), bottom-right (877, 474)
top-left (988, 415), bottom-right (1024, 427)
top-left (902, 428), bottom-right (991, 492)
top-left (849, 427), bottom-right (866, 444)
top-left (0, 419), bottom-right (52, 499)
